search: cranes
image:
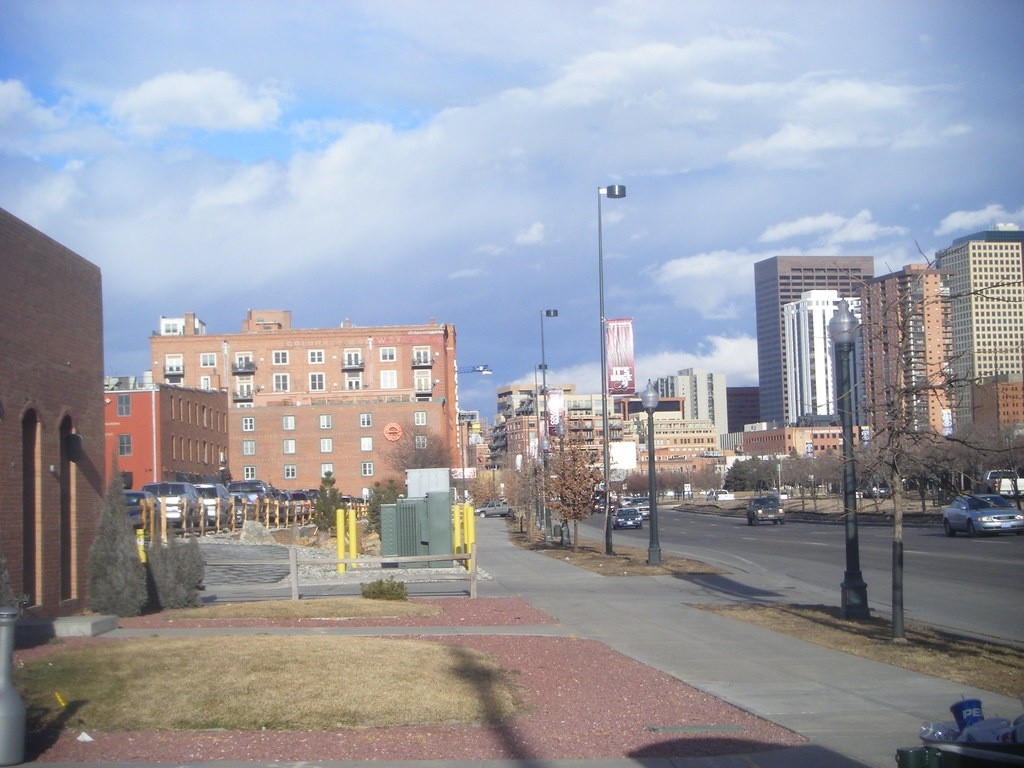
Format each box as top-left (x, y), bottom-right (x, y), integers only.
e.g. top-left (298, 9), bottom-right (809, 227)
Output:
top-left (458, 365), bottom-right (493, 375)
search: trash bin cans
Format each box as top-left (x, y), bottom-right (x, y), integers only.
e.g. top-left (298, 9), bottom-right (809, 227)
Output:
top-left (895, 737), bottom-right (1024, 768)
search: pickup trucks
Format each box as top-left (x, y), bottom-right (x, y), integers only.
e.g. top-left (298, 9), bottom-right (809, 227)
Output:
top-left (982, 468), bottom-right (1024, 495)
top-left (706, 489), bottom-right (734, 501)
top-left (475, 501), bottom-right (514, 518)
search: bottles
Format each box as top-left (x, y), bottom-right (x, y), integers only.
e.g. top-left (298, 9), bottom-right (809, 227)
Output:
top-left (919, 719), bottom-right (959, 741)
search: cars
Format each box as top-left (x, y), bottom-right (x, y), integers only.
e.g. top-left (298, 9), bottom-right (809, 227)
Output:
top-left (612, 508), bottom-right (643, 530)
top-left (593, 497), bottom-right (650, 519)
top-left (943, 494), bottom-right (1024, 538)
top-left (124, 479), bottom-right (365, 534)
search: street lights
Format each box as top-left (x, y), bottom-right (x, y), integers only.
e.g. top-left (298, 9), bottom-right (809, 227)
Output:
top-left (535, 364), bottom-right (547, 459)
top-left (598, 184), bottom-right (627, 556)
top-left (541, 310), bottom-right (558, 436)
top-left (556, 422), bottom-right (571, 545)
top-left (827, 293), bottom-right (871, 620)
top-left (541, 436), bottom-right (553, 541)
top-left (641, 378), bottom-right (661, 566)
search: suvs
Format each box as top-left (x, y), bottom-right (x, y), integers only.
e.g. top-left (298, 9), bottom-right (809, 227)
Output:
top-left (747, 496), bottom-right (785, 525)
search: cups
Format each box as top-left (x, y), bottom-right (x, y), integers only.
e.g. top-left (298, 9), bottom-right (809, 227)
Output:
top-left (950, 698), bottom-right (984, 732)
top-left (996, 728), bottom-right (1024, 743)
